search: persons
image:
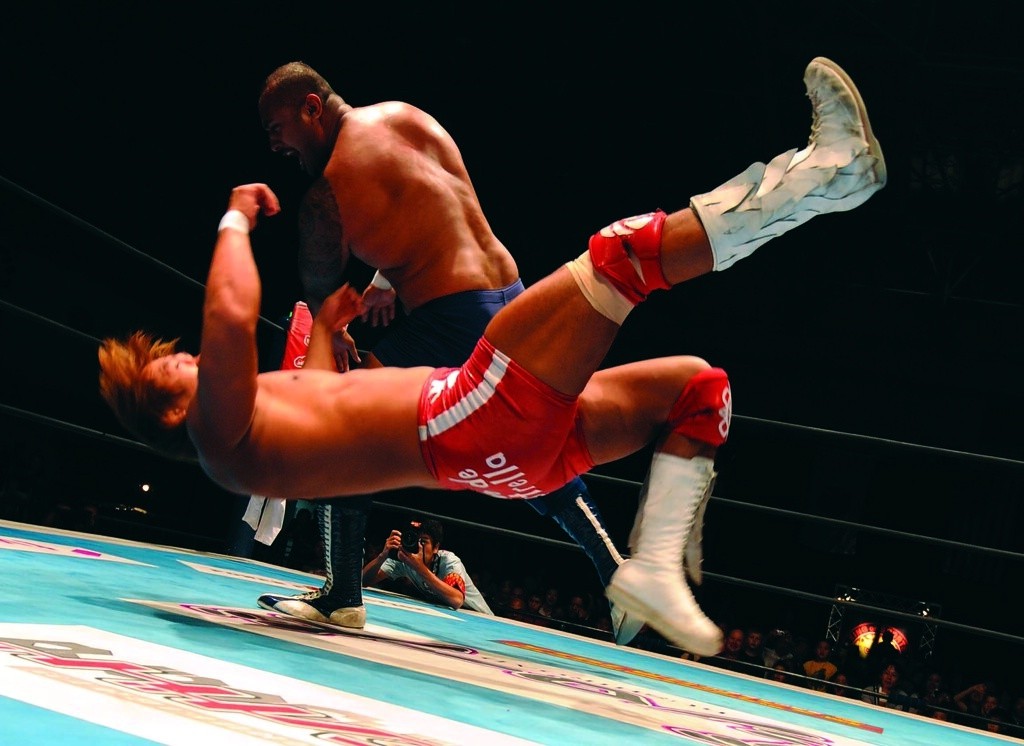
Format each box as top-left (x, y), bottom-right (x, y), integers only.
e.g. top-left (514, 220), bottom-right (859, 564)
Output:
top-left (473, 574), bottom-right (647, 649)
top-left (258, 62), bottom-right (647, 646)
top-left (362, 518), bottom-right (492, 615)
top-left (680, 620), bottom-right (1024, 737)
top-left (97, 58), bottom-right (886, 656)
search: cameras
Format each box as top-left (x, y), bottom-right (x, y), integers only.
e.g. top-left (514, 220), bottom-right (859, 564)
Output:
top-left (393, 528), bottom-right (425, 555)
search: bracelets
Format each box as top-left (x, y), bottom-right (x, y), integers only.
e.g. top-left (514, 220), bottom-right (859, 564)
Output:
top-left (370, 269), bottom-right (391, 291)
top-left (218, 210), bottom-right (250, 234)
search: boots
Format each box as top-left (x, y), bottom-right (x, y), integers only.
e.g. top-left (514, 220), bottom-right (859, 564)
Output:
top-left (689, 56), bottom-right (887, 271)
top-left (257, 501), bottom-right (366, 633)
top-left (604, 452), bottom-right (725, 658)
top-left (552, 495), bottom-right (645, 646)
